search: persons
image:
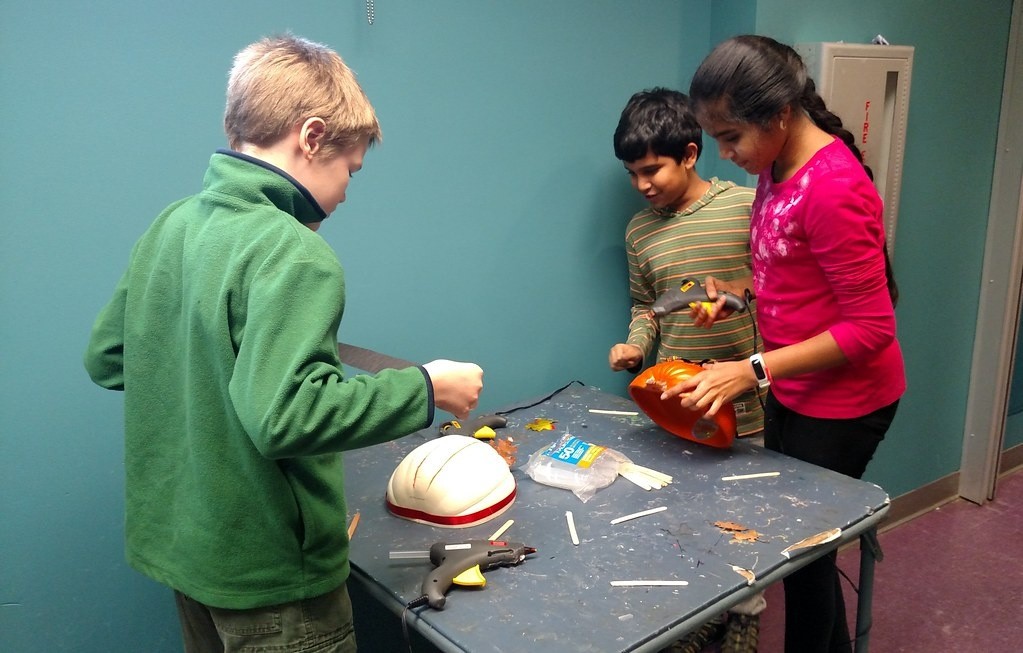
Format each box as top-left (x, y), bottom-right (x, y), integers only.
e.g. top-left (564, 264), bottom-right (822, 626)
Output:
top-left (84, 33), bottom-right (485, 653)
top-left (610, 87), bottom-right (772, 652)
top-left (656, 36), bottom-right (907, 653)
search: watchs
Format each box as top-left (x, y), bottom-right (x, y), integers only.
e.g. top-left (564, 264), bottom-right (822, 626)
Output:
top-left (749, 353), bottom-right (772, 389)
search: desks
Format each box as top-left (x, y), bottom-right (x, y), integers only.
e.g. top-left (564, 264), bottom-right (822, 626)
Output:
top-left (338, 380), bottom-right (894, 653)
